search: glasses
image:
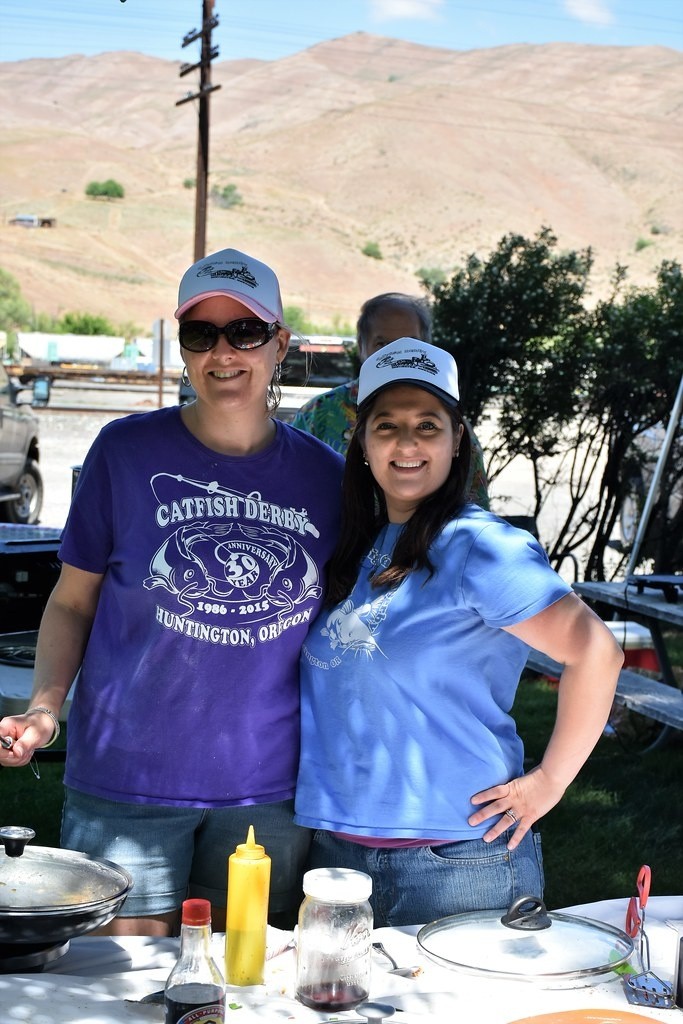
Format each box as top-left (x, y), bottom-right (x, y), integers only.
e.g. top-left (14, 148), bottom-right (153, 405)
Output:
top-left (179, 317), bottom-right (278, 353)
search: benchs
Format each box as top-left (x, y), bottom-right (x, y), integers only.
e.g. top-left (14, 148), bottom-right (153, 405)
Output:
top-left (526, 648), bottom-right (683, 732)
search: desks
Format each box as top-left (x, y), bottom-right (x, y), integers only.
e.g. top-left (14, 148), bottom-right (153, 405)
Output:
top-left (0, 895), bottom-right (683, 1024)
top-left (571, 582), bottom-right (683, 626)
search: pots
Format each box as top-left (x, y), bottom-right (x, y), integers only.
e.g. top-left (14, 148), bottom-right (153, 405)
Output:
top-left (0, 824), bottom-right (134, 945)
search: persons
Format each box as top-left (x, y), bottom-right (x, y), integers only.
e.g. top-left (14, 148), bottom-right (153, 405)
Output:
top-left (293, 290), bottom-right (491, 511)
top-left (0, 249), bottom-right (352, 936)
top-left (302, 348), bottom-right (625, 928)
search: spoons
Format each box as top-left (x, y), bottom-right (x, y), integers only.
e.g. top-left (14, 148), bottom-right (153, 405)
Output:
top-left (371, 943), bottom-right (420, 975)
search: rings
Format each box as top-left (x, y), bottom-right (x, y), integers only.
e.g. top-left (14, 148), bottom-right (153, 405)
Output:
top-left (505, 810), bottom-right (519, 823)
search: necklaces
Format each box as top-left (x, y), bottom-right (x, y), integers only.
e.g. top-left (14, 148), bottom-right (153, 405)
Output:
top-left (367, 521), bottom-right (405, 579)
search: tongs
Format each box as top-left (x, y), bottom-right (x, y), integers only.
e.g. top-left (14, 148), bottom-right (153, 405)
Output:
top-left (625, 864), bottom-right (675, 1009)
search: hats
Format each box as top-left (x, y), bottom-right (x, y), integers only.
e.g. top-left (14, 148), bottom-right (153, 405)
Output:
top-left (357, 336), bottom-right (461, 416)
top-left (174, 248), bottom-right (283, 324)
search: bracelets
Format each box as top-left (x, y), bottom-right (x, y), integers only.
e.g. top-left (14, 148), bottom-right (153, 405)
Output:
top-left (25, 707), bottom-right (61, 749)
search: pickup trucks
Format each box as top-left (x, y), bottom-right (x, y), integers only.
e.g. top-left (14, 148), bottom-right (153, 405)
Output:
top-left (0, 406), bottom-right (43, 525)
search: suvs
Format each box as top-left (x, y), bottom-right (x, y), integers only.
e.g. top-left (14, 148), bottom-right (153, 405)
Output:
top-left (267, 335), bottom-right (357, 424)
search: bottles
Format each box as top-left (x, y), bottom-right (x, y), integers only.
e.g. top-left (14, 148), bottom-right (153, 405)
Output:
top-left (163, 900), bottom-right (226, 1024)
top-left (298, 868), bottom-right (374, 1011)
top-left (225, 825), bottom-right (271, 985)
